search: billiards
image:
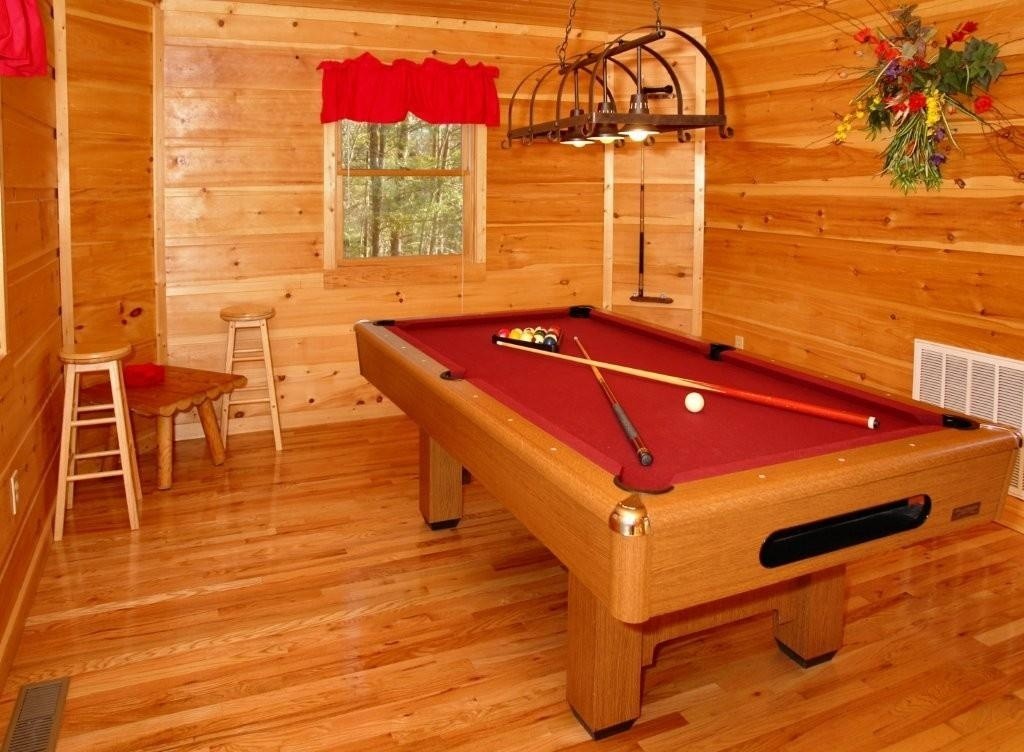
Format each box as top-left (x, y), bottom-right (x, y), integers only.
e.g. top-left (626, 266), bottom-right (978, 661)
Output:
top-left (497, 325), bottom-right (560, 345)
top-left (684, 391), bottom-right (705, 413)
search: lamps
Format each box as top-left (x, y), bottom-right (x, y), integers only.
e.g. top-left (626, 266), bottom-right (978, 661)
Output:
top-left (502, 1), bottom-right (736, 152)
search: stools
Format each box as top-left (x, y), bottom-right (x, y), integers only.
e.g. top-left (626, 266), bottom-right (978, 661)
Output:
top-left (54, 340), bottom-right (145, 544)
top-left (219, 301), bottom-right (285, 455)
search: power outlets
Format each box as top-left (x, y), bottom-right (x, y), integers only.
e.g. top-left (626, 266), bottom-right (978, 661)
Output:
top-left (10, 468), bottom-right (23, 515)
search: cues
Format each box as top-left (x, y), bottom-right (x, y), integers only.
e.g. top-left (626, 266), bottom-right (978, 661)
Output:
top-left (496, 340), bottom-right (880, 430)
top-left (639, 73), bottom-right (645, 297)
top-left (573, 335), bottom-right (654, 466)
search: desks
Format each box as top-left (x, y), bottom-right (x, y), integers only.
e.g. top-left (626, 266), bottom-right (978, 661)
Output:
top-left (353, 297), bottom-right (1021, 740)
top-left (80, 362), bottom-right (249, 492)
